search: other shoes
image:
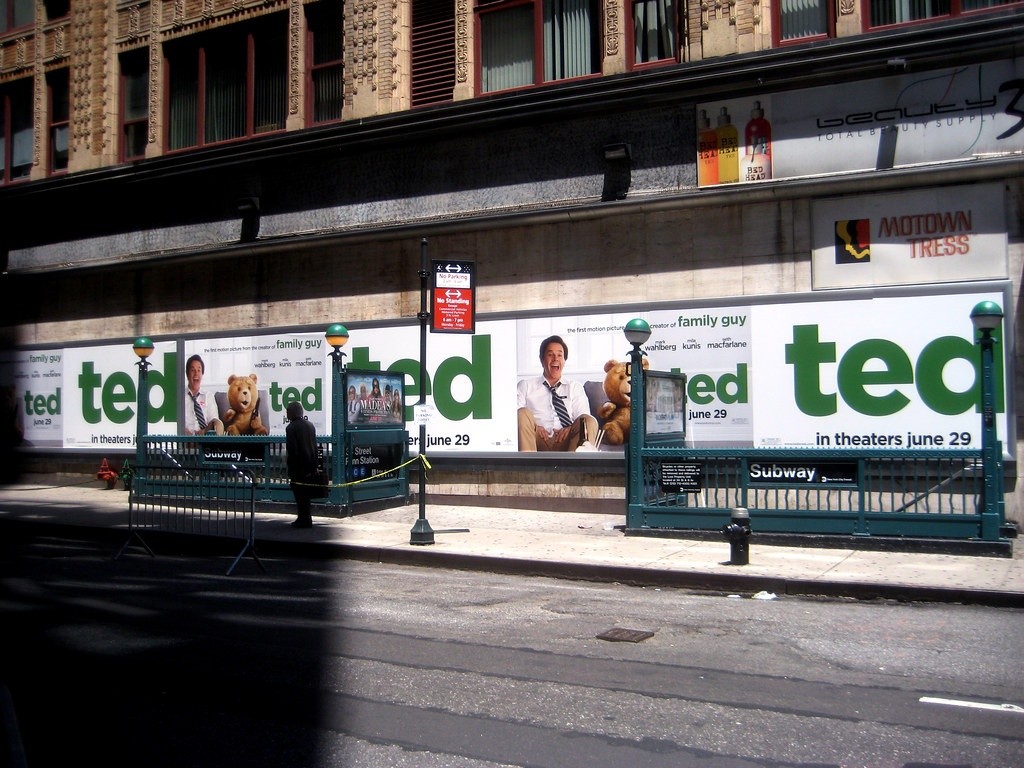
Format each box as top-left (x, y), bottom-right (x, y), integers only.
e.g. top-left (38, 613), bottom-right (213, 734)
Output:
top-left (290, 519), bottom-right (312, 528)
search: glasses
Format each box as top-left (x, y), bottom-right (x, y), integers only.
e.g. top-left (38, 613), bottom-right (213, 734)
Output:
top-left (373, 384), bottom-right (378, 387)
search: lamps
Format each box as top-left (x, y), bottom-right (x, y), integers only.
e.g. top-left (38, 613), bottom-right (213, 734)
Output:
top-left (603, 142), bottom-right (634, 162)
top-left (236, 197), bottom-right (261, 215)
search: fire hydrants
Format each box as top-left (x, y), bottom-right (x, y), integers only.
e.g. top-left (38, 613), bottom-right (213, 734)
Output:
top-left (720, 507), bottom-right (754, 565)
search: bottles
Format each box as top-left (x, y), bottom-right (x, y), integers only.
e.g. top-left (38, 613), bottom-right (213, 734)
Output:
top-left (577, 418), bottom-right (589, 446)
top-left (696, 110), bottom-right (719, 185)
top-left (207, 421), bottom-right (217, 436)
top-left (745, 99), bottom-right (772, 182)
top-left (251, 398), bottom-right (260, 419)
top-left (716, 106), bottom-right (739, 183)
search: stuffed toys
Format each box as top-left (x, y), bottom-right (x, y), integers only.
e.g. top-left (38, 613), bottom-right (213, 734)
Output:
top-left (222, 374), bottom-right (268, 435)
top-left (601, 358), bottom-right (649, 444)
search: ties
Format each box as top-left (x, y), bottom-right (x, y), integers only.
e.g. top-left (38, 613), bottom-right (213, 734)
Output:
top-left (544, 381), bottom-right (572, 427)
top-left (188, 392), bottom-right (208, 429)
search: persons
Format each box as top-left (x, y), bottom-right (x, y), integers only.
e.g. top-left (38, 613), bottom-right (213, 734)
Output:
top-left (185, 354), bottom-right (218, 436)
top-left (285, 401), bottom-right (319, 526)
top-left (348, 378), bottom-right (401, 423)
top-left (517, 335), bottom-right (599, 452)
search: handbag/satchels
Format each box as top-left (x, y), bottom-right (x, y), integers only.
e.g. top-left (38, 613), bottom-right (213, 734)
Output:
top-left (307, 474), bottom-right (329, 498)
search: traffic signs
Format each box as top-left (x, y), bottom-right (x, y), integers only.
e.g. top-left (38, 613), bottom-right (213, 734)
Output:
top-left (432, 260), bottom-right (475, 332)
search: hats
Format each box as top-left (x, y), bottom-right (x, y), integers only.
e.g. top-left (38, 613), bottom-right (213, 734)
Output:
top-left (386, 385), bottom-right (391, 392)
